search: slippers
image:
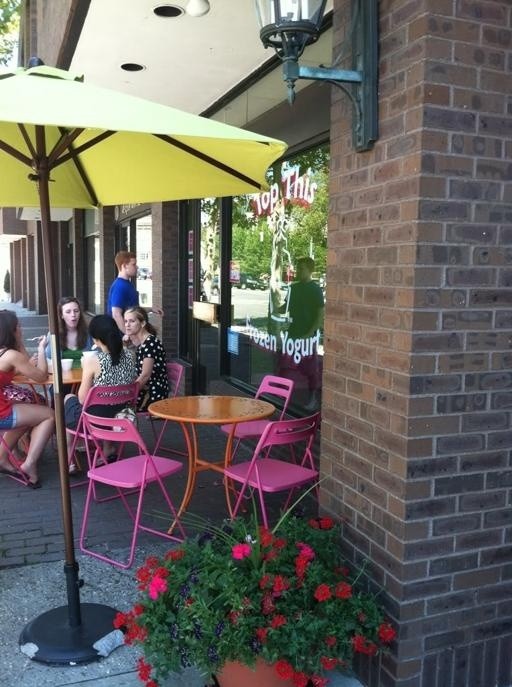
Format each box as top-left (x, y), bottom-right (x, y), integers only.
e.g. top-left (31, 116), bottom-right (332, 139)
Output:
top-left (0, 468), bottom-right (15, 477)
top-left (17, 465), bottom-right (41, 489)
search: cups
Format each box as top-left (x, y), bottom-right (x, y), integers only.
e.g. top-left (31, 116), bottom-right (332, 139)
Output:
top-left (80, 351), bottom-right (98, 356)
top-left (61, 358), bottom-right (74, 371)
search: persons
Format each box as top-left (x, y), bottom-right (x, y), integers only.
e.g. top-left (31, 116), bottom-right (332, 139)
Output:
top-left (270, 257), bottom-right (325, 414)
top-left (45, 296), bottom-right (95, 409)
top-left (64, 316), bottom-right (138, 475)
top-left (122, 305), bottom-right (171, 412)
top-left (107, 249), bottom-right (165, 348)
top-left (1, 309), bottom-right (55, 489)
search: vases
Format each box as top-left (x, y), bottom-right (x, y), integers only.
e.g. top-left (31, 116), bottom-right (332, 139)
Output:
top-left (202, 603), bottom-right (301, 685)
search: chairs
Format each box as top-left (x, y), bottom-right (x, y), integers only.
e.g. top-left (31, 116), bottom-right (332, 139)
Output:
top-left (64, 381), bottom-right (147, 502)
top-left (220, 375), bottom-right (294, 500)
top-left (223, 413), bottom-right (320, 533)
top-left (1, 427), bottom-right (33, 486)
top-left (79, 411), bottom-right (189, 568)
top-left (115, 362), bottom-right (184, 458)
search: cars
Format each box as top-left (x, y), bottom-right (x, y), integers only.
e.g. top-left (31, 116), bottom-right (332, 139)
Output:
top-left (134, 268), bottom-right (152, 280)
top-left (210, 269), bottom-right (269, 298)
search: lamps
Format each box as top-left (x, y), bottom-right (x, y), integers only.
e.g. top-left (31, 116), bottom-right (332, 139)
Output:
top-left (248, 0), bottom-right (381, 151)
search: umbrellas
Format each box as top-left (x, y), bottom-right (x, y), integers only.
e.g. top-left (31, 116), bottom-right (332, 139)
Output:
top-left (1, 55), bottom-right (290, 626)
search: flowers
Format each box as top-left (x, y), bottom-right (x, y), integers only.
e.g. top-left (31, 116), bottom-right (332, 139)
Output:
top-left (112, 517), bottom-right (399, 686)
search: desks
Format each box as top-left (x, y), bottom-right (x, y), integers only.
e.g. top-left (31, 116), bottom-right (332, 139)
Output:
top-left (147, 396), bottom-right (275, 537)
top-left (10, 368), bottom-right (84, 454)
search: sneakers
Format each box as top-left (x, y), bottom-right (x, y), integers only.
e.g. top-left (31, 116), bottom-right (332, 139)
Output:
top-left (68, 459), bottom-right (80, 474)
top-left (95, 454), bottom-right (103, 466)
